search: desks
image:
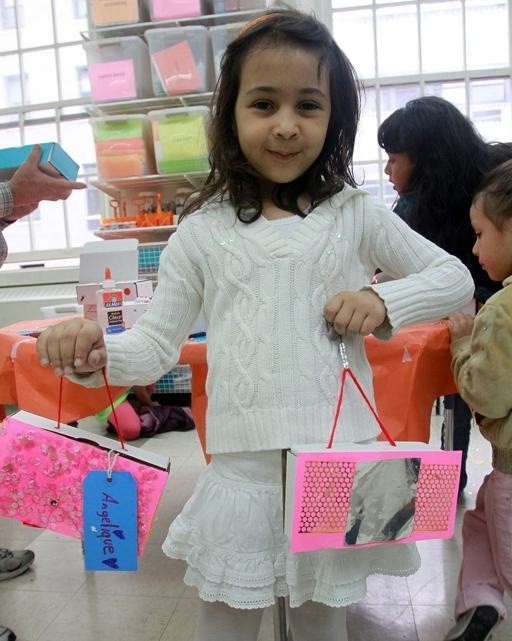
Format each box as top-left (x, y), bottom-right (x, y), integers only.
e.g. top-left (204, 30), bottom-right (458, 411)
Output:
top-left (2, 307), bottom-right (463, 463)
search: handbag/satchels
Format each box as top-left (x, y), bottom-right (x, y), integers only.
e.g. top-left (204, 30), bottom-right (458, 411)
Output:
top-left (273, 369), bottom-right (462, 548)
top-left (1, 365), bottom-right (173, 574)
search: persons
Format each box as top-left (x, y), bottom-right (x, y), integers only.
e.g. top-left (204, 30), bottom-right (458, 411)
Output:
top-left (376, 98), bottom-right (510, 640)
top-left (0, 143), bottom-right (87, 641)
top-left (36, 10), bottom-right (475, 641)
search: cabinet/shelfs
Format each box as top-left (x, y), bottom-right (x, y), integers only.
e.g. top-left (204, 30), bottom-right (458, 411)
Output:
top-left (79, 2), bottom-right (289, 240)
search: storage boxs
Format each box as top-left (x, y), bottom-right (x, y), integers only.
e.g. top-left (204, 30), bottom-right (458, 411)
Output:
top-left (87, 1), bottom-right (264, 178)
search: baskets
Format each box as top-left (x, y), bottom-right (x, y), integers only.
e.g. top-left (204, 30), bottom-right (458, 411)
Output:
top-left (139, 241), bottom-right (168, 275)
top-left (153, 363), bottom-right (193, 394)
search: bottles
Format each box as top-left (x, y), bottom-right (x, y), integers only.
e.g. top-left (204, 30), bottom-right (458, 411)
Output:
top-left (95, 268), bottom-right (126, 338)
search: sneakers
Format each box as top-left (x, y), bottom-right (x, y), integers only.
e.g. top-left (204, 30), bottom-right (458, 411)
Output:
top-left (445, 604), bottom-right (500, 637)
top-left (2, 547), bottom-right (34, 581)
